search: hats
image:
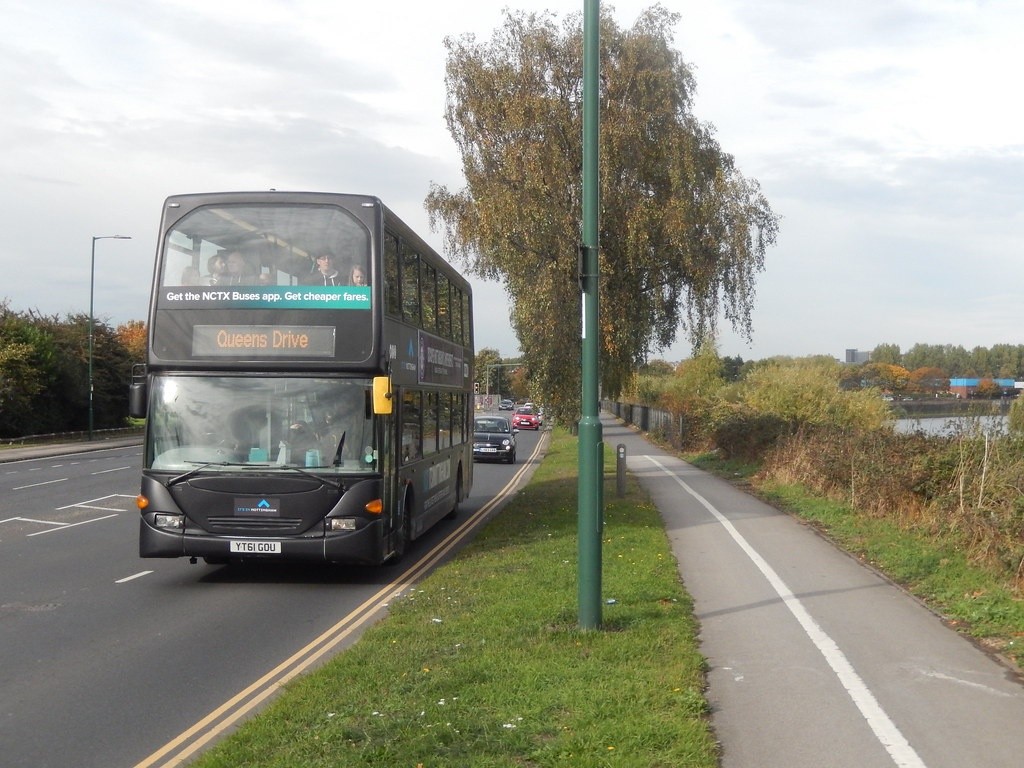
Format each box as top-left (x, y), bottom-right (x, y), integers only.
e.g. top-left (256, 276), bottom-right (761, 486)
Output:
top-left (314, 246), bottom-right (335, 263)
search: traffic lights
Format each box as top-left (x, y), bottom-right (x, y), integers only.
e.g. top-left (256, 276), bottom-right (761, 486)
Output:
top-left (474, 382), bottom-right (480, 395)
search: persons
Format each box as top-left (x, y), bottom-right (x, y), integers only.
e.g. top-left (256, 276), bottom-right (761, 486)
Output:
top-left (198, 248), bottom-right (368, 285)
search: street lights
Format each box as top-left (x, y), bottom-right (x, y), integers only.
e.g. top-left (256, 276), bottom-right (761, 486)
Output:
top-left (85, 234), bottom-right (133, 441)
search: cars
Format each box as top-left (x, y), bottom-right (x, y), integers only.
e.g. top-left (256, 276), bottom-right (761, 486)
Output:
top-left (498, 400), bottom-right (515, 411)
top-left (473, 416), bottom-right (520, 464)
top-left (512, 406), bottom-right (539, 430)
top-left (521, 403), bottom-right (544, 426)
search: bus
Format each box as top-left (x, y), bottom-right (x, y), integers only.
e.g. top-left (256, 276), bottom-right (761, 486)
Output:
top-left (128, 188), bottom-right (475, 566)
